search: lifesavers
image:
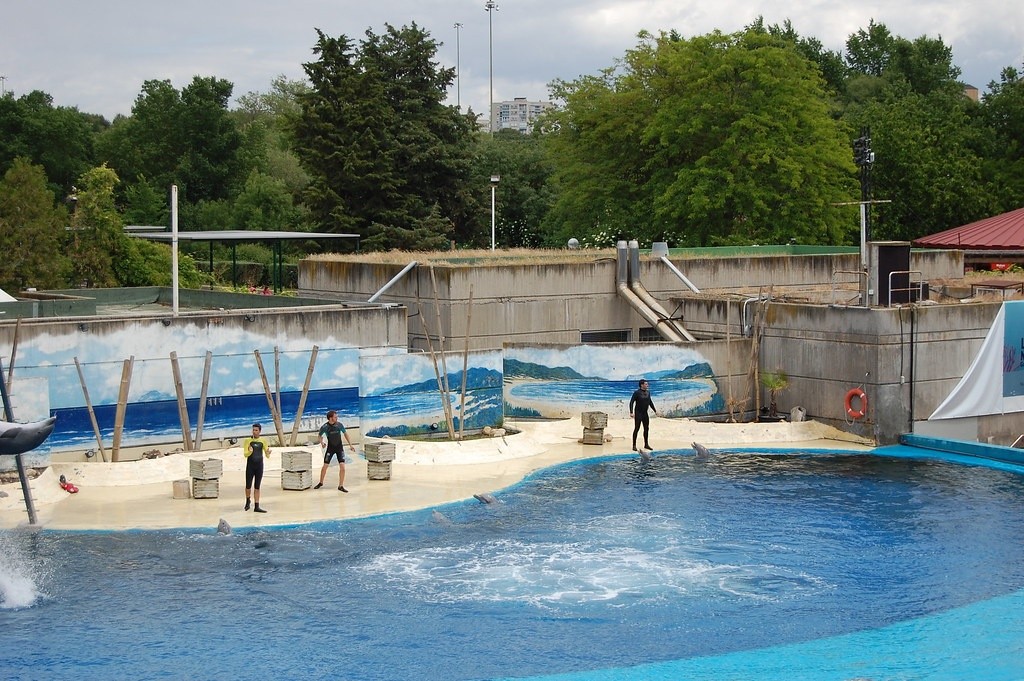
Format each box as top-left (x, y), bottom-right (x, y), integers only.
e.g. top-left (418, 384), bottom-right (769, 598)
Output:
top-left (846, 388), bottom-right (867, 419)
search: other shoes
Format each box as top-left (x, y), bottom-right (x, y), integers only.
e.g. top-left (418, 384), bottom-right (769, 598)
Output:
top-left (314, 483), bottom-right (323, 489)
top-left (633, 447), bottom-right (637, 451)
top-left (254, 508), bottom-right (267, 512)
top-left (338, 486), bottom-right (348, 492)
top-left (644, 445), bottom-right (653, 450)
top-left (245, 501), bottom-right (251, 511)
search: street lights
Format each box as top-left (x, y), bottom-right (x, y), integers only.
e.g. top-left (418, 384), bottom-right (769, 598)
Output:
top-left (454, 22), bottom-right (464, 107)
top-left (484, 0), bottom-right (500, 136)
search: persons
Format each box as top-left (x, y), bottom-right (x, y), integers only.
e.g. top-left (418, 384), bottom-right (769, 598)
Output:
top-left (314, 411), bottom-right (355, 492)
top-left (250, 285), bottom-right (258, 293)
top-left (263, 286), bottom-right (272, 295)
top-left (629, 380), bottom-right (660, 452)
top-left (243, 423), bottom-right (272, 512)
